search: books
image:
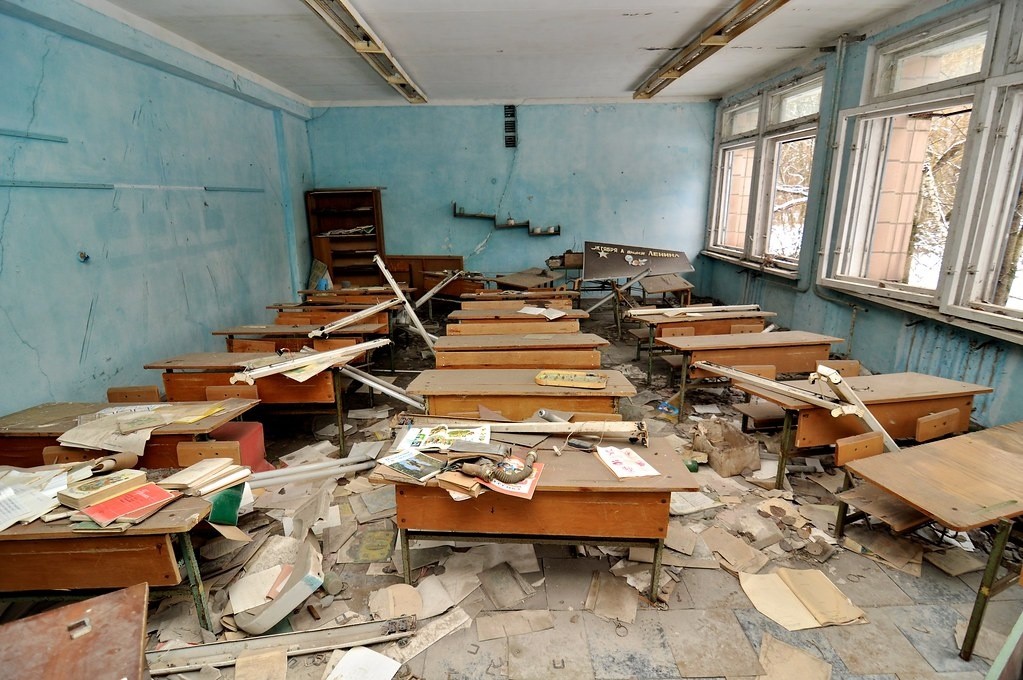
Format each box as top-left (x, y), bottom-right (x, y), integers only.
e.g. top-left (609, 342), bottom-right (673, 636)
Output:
top-left (670, 491), bottom-right (870, 631)
top-left (71, 483), bottom-right (183, 532)
top-left (119, 398), bottom-right (262, 434)
top-left (57, 468), bottom-right (146, 510)
top-left (377, 425), bottom-right (507, 498)
top-left (0, 485), bottom-right (76, 531)
top-left (518, 306), bottom-right (568, 318)
top-left (158, 457), bottom-right (255, 497)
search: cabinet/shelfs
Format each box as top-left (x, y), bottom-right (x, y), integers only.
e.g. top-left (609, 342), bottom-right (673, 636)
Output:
top-left (308, 188), bottom-right (463, 304)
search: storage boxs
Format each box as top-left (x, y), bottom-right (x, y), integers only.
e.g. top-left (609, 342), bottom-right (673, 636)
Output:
top-left (563, 253), bottom-right (582, 267)
top-left (548, 260), bottom-right (562, 267)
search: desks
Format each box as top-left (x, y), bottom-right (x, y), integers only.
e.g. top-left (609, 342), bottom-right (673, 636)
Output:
top-left (1, 266), bottom-right (1023, 658)
